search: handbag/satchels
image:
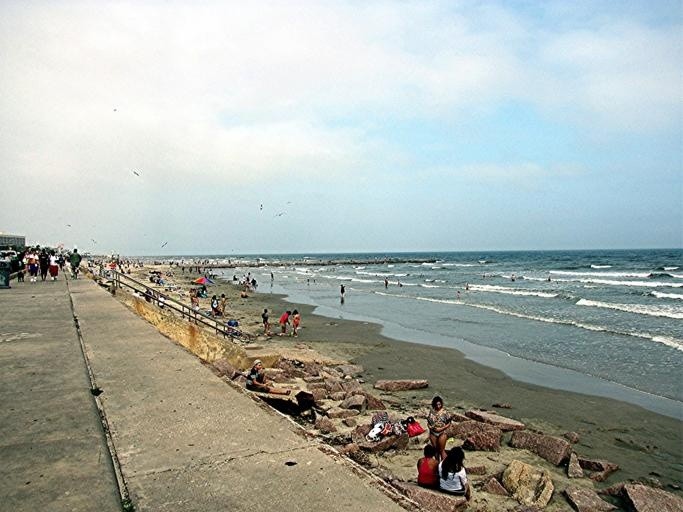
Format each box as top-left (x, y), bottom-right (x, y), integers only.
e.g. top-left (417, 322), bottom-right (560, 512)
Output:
top-left (403, 416), bottom-right (424, 436)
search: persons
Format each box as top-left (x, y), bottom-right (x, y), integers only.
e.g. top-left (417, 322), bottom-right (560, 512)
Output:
top-left (228, 318), bottom-right (241, 331)
top-left (175, 287), bottom-right (226, 316)
top-left (427, 397), bottom-right (451, 459)
top-left (384, 276), bottom-right (389, 289)
top-left (134, 287), bottom-right (164, 308)
top-left (340, 284), bottom-right (345, 298)
top-left (233, 272), bottom-right (256, 298)
top-left (457, 291), bottom-right (461, 300)
top-left (15, 243), bottom-right (82, 282)
top-left (87, 253), bottom-right (144, 280)
top-left (304, 270), bottom-right (309, 282)
top-left (270, 272), bottom-right (273, 279)
top-left (397, 279), bottom-right (403, 287)
top-left (417, 445), bottom-right (440, 489)
top-left (279, 309), bottom-right (301, 335)
top-left (262, 308), bottom-right (271, 332)
top-left (149, 259), bottom-right (212, 285)
top-left (438, 447), bottom-right (470, 500)
top-left (465, 283), bottom-right (470, 292)
top-left (246, 359), bottom-right (292, 395)
